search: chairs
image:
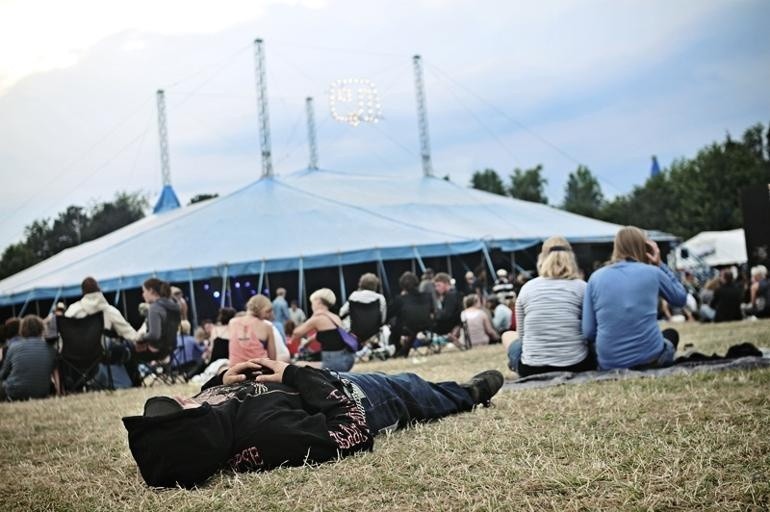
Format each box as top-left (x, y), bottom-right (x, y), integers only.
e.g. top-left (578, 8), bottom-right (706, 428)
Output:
top-left (347, 297), bottom-right (384, 361)
top-left (120, 307), bottom-right (190, 384)
top-left (429, 294), bottom-right (473, 351)
top-left (393, 292), bottom-right (438, 358)
top-left (54, 309), bottom-right (116, 396)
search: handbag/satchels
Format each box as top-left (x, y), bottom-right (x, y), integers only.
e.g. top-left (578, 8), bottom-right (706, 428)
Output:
top-left (338, 326), bottom-right (358, 351)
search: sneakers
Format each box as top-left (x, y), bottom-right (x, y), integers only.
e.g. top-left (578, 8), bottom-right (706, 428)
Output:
top-left (460, 370), bottom-right (504, 407)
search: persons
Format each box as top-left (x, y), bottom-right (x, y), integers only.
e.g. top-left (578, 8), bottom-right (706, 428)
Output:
top-left (592, 258), bottom-right (602, 270)
top-left (120, 356), bottom-right (504, 489)
top-left (500, 235), bottom-right (598, 378)
top-left (1, 270), bottom-right (532, 405)
top-left (657, 249), bottom-right (770, 323)
top-left (580, 224), bottom-right (689, 371)
